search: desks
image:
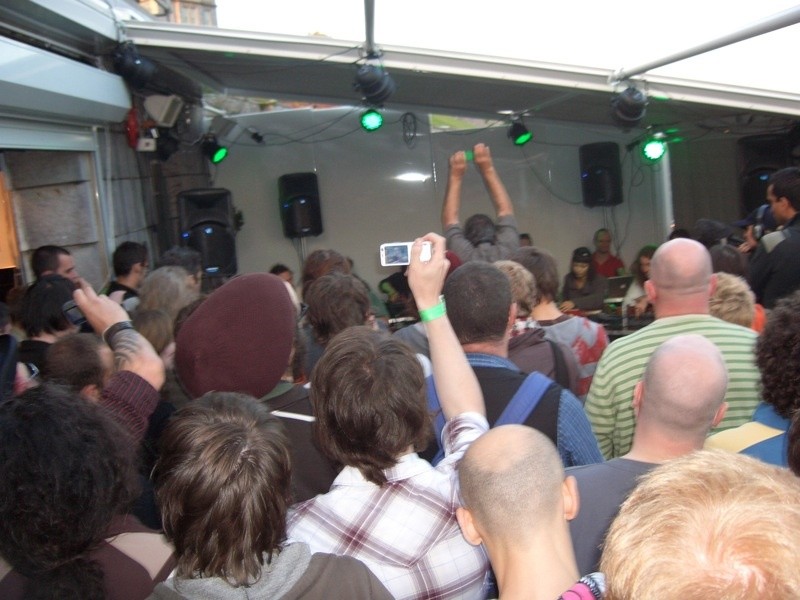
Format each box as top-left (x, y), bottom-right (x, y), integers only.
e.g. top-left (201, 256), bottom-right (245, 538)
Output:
top-left (585, 313), bottom-right (658, 344)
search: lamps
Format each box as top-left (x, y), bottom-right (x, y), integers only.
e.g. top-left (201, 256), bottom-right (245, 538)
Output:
top-left (511, 122), bottom-right (532, 146)
top-left (608, 87), bottom-right (647, 129)
top-left (143, 93), bottom-right (183, 127)
top-left (113, 47), bottom-right (158, 89)
top-left (360, 109), bottom-right (383, 133)
top-left (354, 64), bottom-right (395, 107)
top-left (201, 140), bottom-right (227, 164)
top-left (642, 137), bottom-right (666, 162)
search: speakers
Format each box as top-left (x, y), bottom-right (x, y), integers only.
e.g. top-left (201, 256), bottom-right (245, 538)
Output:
top-left (278, 172), bottom-right (324, 239)
top-left (579, 141), bottom-right (623, 207)
top-left (177, 189), bottom-right (238, 279)
top-left (738, 134), bottom-right (791, 219)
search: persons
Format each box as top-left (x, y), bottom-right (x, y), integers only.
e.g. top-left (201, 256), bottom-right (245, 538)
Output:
top-left (0, 141), bottom-right (800, 599)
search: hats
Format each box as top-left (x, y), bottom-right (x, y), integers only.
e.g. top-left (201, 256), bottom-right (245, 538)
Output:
top-left (573, 246), bottom-right (591, 263)
top-left (173, 272), bottom-right (297, 402)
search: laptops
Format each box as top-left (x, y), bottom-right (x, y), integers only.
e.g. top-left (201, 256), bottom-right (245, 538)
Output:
top-left (608, 275), bottom-right (634, 299)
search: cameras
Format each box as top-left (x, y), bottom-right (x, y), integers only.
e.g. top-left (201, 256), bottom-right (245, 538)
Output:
top-left (380, 241), bottom-right (433, 266)
top-left (61, 300), bottom-right (88, 326)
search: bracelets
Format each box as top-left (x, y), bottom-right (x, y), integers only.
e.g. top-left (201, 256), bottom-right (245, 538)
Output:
top-left (103, 321), bottom-right (136, 348)
top-left (418, 294), bottom-right (448, 321)
